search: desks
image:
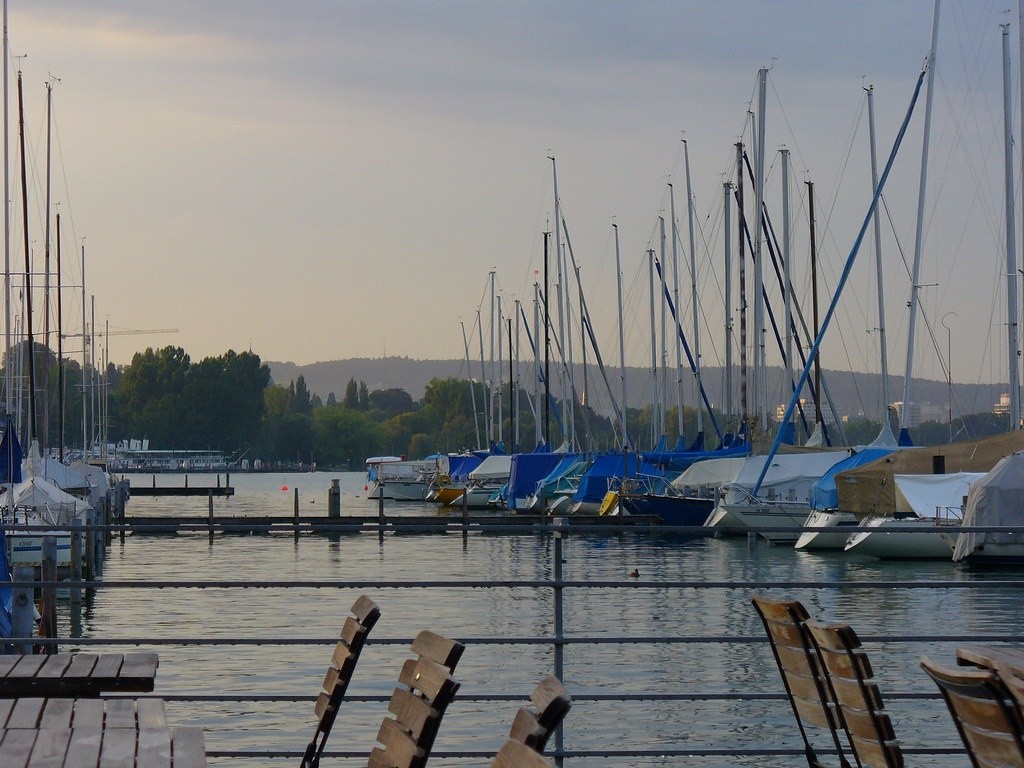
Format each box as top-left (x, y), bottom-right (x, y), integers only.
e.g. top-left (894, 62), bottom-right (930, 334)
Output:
top-left (0, 653), bottom-right (159, 696)
top-left (1, 696), bottom-right (204, 768)
top-left (956, 649), bottom-right (1024, 704)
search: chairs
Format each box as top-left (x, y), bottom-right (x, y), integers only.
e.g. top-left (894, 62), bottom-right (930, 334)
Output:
top-left (803, 617), bottom-right (905, 768)
top-left (918, 654), bottom-right (1024, 768)
top-left (751, 593), bottom-right (855, 768)
top-left (206, 594), bottom-right (571, 768)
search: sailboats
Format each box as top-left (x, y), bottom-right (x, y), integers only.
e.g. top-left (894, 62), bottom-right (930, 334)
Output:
top-left (0, 0), bottom-right (133, 656)
top-left (366, 1), bottom-right (1023, 564)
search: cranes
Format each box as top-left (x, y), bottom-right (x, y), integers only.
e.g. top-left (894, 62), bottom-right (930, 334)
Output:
top-left (56, 324), bottom-right (181, 440)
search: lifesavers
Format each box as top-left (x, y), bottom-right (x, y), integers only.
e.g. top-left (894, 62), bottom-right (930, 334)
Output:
top-left (438, 475), bottom-right (451, 488)
